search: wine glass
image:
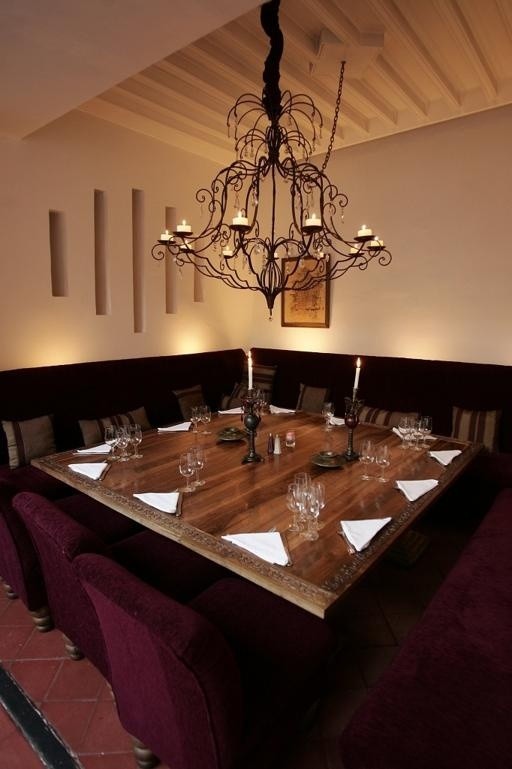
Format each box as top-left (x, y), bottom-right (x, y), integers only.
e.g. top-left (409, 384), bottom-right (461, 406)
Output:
top-left (283, 470), bottom-right (329, 542)
top-left (188, 403), bottom-right (214, 435)
top-left (396, 413), bottom-right (433, 452)
top-left (103, 423), bottom-right (145, 463)
top-left (319, 401), bottom-right (336, 433)
top-left (357, 437), bottom-right (392, 484)
top-left (177, 444), bottom-right (207, 494)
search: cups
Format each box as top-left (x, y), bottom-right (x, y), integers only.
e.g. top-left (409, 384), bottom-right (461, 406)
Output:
top-left (285, 428), bottom-right (296, 448)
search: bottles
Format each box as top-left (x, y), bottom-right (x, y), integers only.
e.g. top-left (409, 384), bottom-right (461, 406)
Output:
top-left (273, 434), bottom-right (282, 454)
top-left (267, 432), bottom-right (274, 455)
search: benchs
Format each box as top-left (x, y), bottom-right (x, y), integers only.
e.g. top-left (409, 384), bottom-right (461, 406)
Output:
top-left (251, 347), bottom-right (509, 475)
top-left (361, 487), bottom-right (511, 693)
top-left (1, 349), bottom-right (246, 486)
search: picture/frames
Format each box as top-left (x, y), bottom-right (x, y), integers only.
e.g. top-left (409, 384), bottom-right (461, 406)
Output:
top-left (281, 254), bottom-right (331, 329)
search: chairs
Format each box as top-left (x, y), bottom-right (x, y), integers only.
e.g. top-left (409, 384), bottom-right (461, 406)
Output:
top-left (2, 483), bottom-right (141, 632)
top-left (71, 556), bottom-right (332, 768)
top-left (9, 493), bottom-right (210, 663)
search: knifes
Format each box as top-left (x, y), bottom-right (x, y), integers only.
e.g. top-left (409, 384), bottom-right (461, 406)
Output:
top-left (280, 532), bottom-right (293, 568)
top-left (175, 487), bottom-right (184, 517)
top-left (98, 463), bottom-right (112, 481)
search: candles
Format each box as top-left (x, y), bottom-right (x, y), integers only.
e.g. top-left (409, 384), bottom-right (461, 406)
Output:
top-left (159, 212), bottom-right (382, 264)
top-left (248, 358), bottom-right (254, 390)
top-left (354, 366), bottom-right (361, 390)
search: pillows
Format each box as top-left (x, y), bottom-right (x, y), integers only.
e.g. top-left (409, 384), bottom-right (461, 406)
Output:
top-left (1, 415), bottom-right (56, 469)
top-left (451, 407), bottom-right (500, 451)
top-left (236, 362), bottom-right (275, 405)
top-left (78, 407), bottom-right (150, 447)
top-left (357, 407), bottom-right (418, 429)
top-left (174, 384), bottom-right (204, 421)
top-left (297, 383), bottom-right (328, 412)
top-left (220, 395), bottom-right (244, 409)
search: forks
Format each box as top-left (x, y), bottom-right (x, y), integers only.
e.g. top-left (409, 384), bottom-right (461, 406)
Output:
top-left (335, 529), bottom-right (356, 554)
